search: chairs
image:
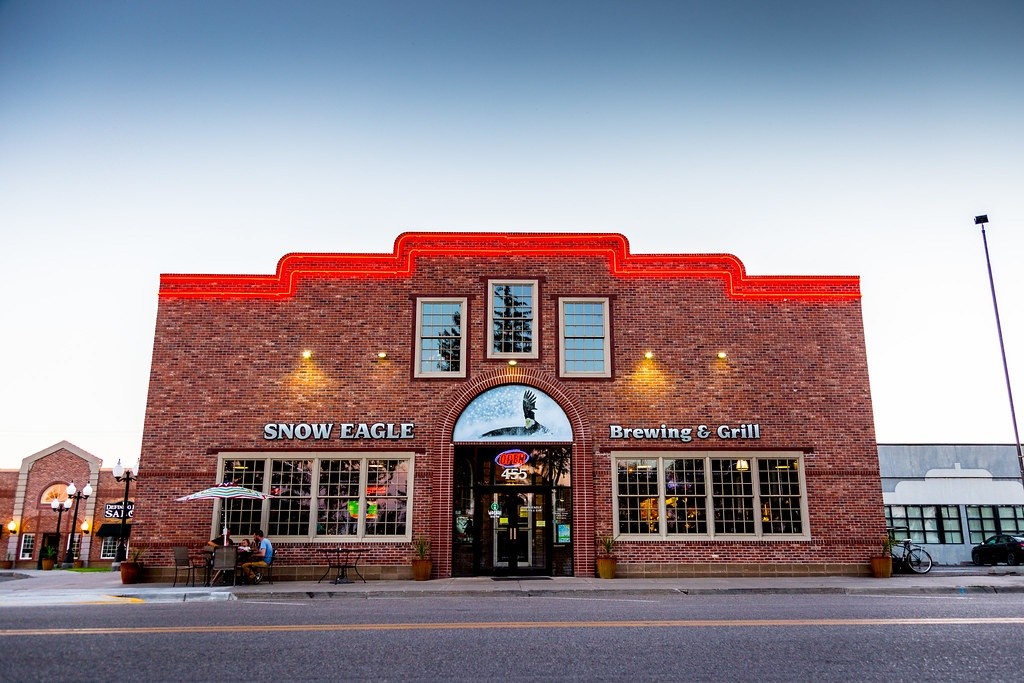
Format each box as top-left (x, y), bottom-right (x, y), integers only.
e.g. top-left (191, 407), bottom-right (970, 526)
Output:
top-left (173, 547), bottom-right (276, 588)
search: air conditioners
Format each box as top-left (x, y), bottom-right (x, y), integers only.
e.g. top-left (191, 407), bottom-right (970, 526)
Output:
top-left (775, 460), bottom-right (791, 468)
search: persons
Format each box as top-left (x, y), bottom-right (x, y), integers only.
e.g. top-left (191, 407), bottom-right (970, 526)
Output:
top-left (208, 528), bottom-right (273, 585)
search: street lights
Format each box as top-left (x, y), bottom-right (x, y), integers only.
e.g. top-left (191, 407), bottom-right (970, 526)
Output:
top-left (62, 480), bottom-right (93, 568)
top-left (111, 458), bottom-right (140, 571)
top-left (51, 496), bottom-right (74, 563)
top-left (974, 214), bottom-right (1024, 487)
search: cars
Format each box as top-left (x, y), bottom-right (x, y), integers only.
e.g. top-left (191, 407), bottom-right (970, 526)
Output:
top-left (971, 534), bottom-right (1024, 566)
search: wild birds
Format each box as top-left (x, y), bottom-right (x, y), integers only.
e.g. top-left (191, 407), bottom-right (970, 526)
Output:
top-left (482, 389), bottom-right (551, 438)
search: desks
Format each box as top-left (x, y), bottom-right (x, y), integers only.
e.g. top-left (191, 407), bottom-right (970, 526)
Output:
top-left (196, 550), bottom-right (253, 585)
top-left (316, 548), bottom-right (370, 584)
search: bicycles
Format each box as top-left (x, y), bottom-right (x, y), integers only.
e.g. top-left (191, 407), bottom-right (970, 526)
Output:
top-left (881, 536), bottom-right (932, 574)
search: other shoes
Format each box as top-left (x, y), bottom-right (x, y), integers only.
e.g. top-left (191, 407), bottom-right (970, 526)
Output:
top-left (253, 576), bottom-right (261, 584)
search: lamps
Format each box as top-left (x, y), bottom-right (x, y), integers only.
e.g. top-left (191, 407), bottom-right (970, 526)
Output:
top-left (736, 459), bottom-right (749, 471)
top-left (8, 519), bottom-right (16, 534)
top-left (81, 520), bottom-right (89, 534)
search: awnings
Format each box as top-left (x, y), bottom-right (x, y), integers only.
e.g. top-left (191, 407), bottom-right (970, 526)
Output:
top-left (95, 523), bottom-right (132, 538)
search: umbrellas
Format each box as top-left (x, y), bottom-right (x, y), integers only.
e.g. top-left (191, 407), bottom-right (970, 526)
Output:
top-left (174, 482), bottom-right (275, 586)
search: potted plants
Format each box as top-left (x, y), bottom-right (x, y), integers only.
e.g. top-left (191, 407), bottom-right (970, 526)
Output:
top-left (1, 554), bottom-right (14, 569)
top-left (120, 537), bottom-right (152, 585)
top-left (74, 552), bottom-right (84, 568)
top-left (412, 536), bottom-right (433, 581)
top-left (42, 546), bottom-right (56, 570)
top-left (870, 537), bottom-right (901, 579)
top-left (596, 533), bottom-right (621, 579)
